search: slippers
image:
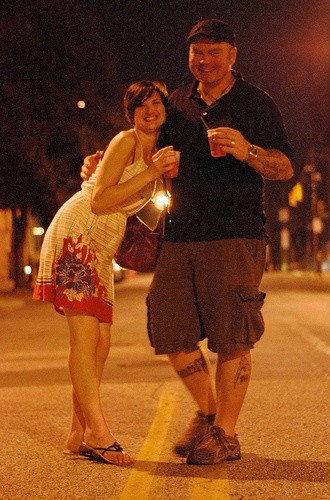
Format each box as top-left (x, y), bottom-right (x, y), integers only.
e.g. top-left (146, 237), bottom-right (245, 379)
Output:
top-left (63, 449), bottom-right (95, 458)
top-left (81, 441), bottom-right (135, 465)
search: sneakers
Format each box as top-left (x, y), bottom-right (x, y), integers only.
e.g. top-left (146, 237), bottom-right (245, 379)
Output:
top-left (173, 412), bottom-right (216, 457)
top-left (187, 425), bottom-right (240, 465)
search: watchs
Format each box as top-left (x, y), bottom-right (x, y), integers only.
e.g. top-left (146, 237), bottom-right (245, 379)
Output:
top-left (245, 143), bottom-right (258, 164)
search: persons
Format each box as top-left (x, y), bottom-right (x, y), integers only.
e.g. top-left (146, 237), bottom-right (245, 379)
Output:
top-left (32, 81), bottom-right (178, 465)
top-left (81, 19), bottom-right (302, 463)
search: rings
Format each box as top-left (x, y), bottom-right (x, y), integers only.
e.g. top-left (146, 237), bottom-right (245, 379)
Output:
top-left (232, 140), bottom-right (235, 146)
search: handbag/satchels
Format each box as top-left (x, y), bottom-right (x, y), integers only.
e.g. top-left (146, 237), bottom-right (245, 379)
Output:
top-left (114, 208), bottom-right (168, 272)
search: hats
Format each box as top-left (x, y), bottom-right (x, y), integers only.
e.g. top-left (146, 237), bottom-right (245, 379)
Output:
top-left (186, 19), bottom-right (236, 45)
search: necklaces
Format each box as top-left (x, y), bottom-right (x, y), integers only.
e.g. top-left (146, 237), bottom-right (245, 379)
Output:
top-left (197, 77), bottom-right (235, 102)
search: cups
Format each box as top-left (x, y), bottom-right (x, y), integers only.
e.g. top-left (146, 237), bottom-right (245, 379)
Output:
top-left (163, 151), bottom-right (180, 179)
top-left (207, 129), bottom-right (227, 157)
